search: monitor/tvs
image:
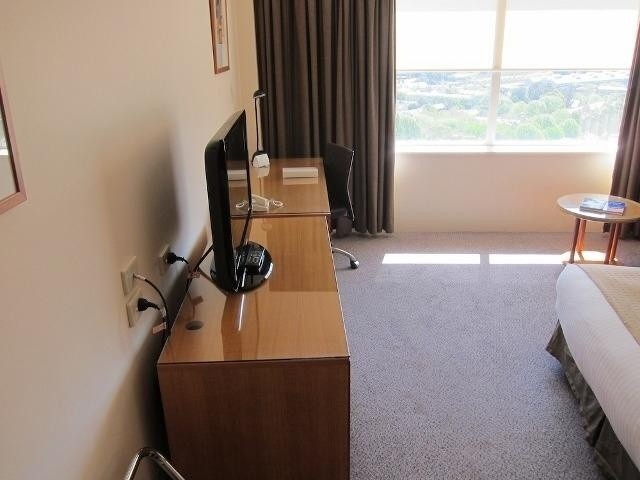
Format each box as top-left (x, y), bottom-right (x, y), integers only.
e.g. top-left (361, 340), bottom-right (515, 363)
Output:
top-left (203, 107), bottom-right (273, 296)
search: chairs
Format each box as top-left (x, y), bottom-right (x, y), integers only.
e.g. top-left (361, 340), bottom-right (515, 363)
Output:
top-left (323, 141), bottom-right (360, 269)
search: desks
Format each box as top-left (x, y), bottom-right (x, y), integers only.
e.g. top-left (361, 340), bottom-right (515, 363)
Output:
top-left (557, 193), bottom-right (640, 264)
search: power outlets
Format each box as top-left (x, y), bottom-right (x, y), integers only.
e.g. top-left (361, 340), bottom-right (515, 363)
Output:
top-left (159, 243), bottom-right (172, 275)
top-left (126, 288), bottom-right (144, 327)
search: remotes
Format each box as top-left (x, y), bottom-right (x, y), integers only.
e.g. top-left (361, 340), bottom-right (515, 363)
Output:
top-left (245, 245), bottom-right (265, 267)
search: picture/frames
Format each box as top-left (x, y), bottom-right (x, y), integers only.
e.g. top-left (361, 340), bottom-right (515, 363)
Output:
top-left (209, 0), bottom-right (230, 74)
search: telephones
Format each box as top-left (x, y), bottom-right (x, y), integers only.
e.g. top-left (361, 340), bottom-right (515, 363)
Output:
top-left (251, 194), bottom-right (283, 212)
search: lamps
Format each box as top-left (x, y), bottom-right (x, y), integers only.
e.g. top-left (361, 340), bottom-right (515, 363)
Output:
top-left (251, 90), bottom-right (270, 167)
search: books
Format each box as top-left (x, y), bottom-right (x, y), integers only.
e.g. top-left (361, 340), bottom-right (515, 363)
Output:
top-left (580, 198), bottom-right (626, 216)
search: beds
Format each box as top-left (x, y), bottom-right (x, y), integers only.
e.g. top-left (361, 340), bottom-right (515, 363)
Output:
top-left (555, 265), bottom-right (640, 480)
